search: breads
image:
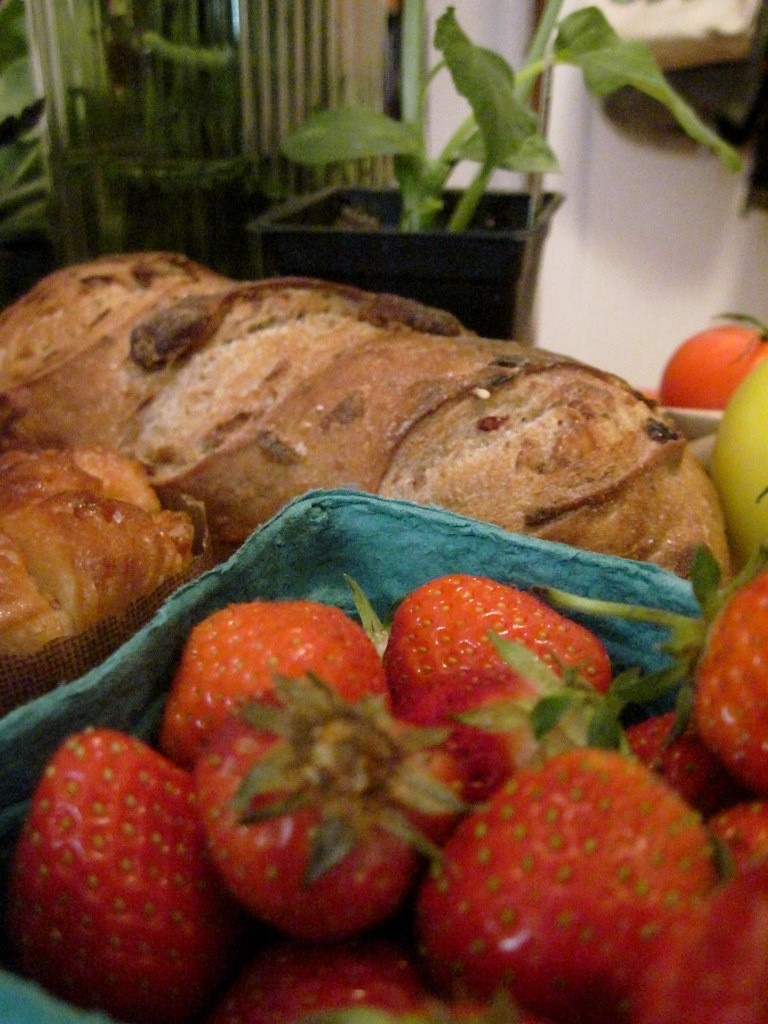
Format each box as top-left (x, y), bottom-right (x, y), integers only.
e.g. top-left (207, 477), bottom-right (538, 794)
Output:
top-left (0, 246), bottom-right (731, 707)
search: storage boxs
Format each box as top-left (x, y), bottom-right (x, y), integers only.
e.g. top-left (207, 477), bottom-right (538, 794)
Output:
top-left (0, 485), bottom-right (700, 1024)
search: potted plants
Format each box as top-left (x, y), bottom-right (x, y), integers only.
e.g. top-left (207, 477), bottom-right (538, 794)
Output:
top-left (241, 0), bottom-right (745, 344)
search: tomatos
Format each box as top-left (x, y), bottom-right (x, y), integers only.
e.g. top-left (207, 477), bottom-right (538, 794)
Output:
top-left (642, 316), bottom-right (768, 577)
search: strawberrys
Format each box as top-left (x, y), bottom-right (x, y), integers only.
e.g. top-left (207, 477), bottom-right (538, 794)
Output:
top-left (9, 546), bottom-right (768, 1024)
